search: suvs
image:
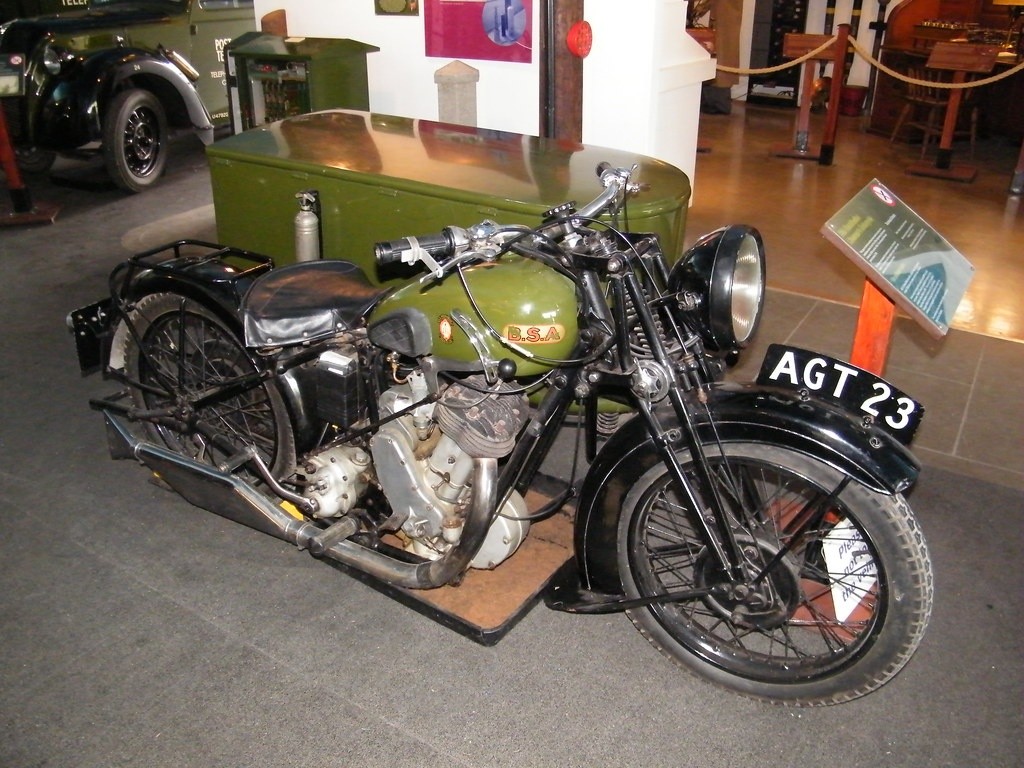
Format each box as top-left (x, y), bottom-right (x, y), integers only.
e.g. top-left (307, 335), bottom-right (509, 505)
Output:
top-left (0, 0), bottom-right (256, 184)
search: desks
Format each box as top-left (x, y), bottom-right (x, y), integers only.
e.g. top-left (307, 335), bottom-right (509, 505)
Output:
top-left (203, 109), bottom-right (692, 417)
top-left (884, 45), bottom-right (1024, 71)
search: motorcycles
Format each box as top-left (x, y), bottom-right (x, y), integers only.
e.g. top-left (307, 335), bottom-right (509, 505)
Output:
top-left (64, 106), bottom-right (932, 709)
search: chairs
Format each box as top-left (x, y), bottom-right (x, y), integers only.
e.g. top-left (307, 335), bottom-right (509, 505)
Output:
top-left (888, 63), bottom-right (980, 151)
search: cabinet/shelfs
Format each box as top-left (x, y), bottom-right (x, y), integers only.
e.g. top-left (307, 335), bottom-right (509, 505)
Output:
top-left (229, 33), bottom-right (380, 132)
